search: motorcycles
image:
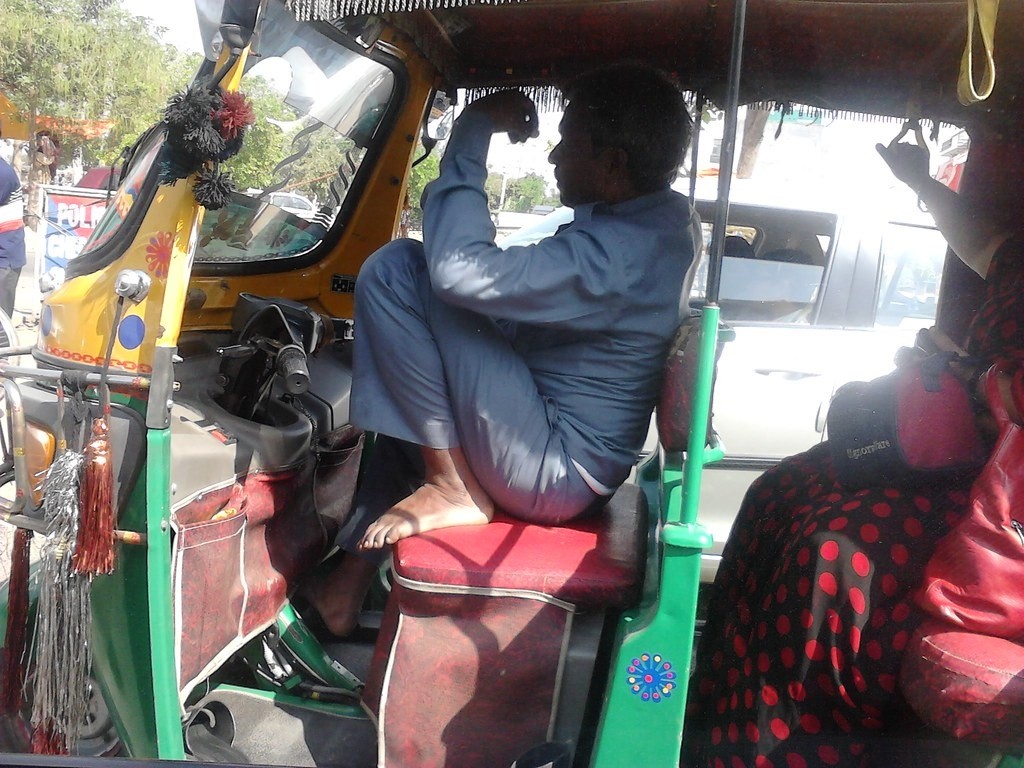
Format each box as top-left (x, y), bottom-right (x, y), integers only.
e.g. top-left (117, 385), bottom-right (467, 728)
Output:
top-left (0, 1), bottom-right (1023, 768)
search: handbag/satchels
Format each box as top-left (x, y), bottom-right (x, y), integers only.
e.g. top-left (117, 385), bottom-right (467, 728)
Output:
top-left (915, 359), bottom-right (1024, 640)
top-left (817, 350), bottom-right (984, 494)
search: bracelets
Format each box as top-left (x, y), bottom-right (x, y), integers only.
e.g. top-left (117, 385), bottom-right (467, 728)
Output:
top-left (917, 177), bottom-right (935, 214)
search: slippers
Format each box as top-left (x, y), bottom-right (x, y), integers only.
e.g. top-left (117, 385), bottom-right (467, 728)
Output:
top-left (181, 700), bottom-right (248, 765)
top-left (288, 568), bottom-right (364, 645)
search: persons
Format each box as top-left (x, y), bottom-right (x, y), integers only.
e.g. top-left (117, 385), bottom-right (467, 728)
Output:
top-left (0, 131), bottom-right (28, 365)
top-left (696, 141), bottom-right (1024, 768)
top-left (296, 60), bottom-right (704, 639)
top-left (741, 247), bottom-right (816, 324)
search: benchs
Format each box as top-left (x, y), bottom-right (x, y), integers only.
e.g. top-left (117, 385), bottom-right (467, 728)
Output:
top-left (871, 615), bottom-right (1024, 768)
top-left (359, 300), bottom-right (724, 768)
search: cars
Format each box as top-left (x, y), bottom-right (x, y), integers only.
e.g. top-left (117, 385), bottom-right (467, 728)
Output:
top-left (496, 176), bottom-right (951, 585)
top-left (75, 166), bottom-right (121, 191)
top-left (246, 188), bottom-right (317, 218)
